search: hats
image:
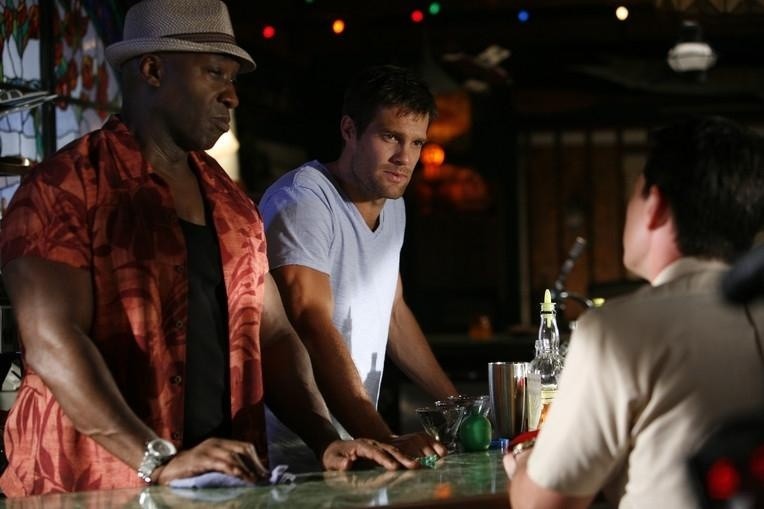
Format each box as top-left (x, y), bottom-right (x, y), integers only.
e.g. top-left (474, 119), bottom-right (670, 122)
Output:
top-left (104, 0), bottom-right (258, 71)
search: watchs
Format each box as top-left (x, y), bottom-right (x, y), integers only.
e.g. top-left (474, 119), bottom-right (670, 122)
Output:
top-left (138, 439), bottom-right (178, 480)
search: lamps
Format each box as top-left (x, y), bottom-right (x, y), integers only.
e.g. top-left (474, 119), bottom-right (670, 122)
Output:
top-left (667, 19), bottom-right (717, 87)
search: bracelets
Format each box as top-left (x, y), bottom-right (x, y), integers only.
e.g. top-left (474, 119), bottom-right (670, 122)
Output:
top-left (511, 441), bottom-right (535, 456)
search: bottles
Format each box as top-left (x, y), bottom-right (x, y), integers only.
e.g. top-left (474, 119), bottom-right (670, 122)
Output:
top-left (519, 286), bottom-right (567, 434)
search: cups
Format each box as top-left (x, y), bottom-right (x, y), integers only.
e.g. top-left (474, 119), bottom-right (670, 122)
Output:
top-left (414, 395), bottom-right (491, 455)
top-left (487, 359), bottom-right (535, 442)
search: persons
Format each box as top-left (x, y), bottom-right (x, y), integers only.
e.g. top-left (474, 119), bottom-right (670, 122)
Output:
top-left (260, 65), bottom-right (460, 467)
top-left (502, 119), bottom-right (764, 509)
top-left (325, 468), bottom-right (412, 501)
top-left (0, 1), bottom-right (416, 509)
